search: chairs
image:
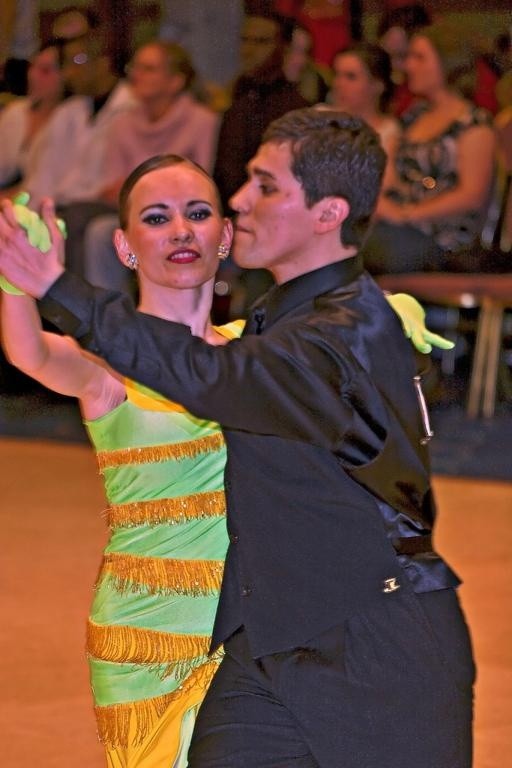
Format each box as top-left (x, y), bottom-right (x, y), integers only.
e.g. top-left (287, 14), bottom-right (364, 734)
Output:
top-left (370, 103), bottom-right (512, 418)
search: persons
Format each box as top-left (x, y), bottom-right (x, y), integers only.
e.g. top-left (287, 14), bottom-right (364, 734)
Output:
top-left (0, 0), bottom-right (511, 421)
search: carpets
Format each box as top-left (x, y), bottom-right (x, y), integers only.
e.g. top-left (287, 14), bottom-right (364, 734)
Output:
top-left (0, 393), bottom-right (512, 485)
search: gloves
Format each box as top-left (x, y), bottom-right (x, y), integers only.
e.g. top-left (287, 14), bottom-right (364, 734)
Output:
top-left (1, 191), bottom-right (67, 297)
top-left (386, 293), bottom-right (454, 353)
